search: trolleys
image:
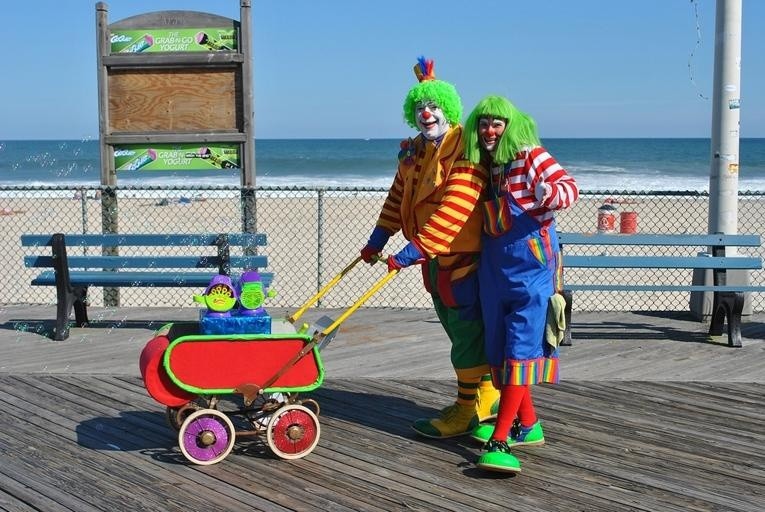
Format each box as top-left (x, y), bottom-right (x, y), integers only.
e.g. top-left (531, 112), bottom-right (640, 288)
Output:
top-left (137, 246), bottom-right (429, 466)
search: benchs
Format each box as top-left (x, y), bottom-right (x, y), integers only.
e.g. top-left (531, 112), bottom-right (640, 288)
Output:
top-left (20, 233), bottom-right (275, 342)
top-left (554, 230), bottom-right (764, 346)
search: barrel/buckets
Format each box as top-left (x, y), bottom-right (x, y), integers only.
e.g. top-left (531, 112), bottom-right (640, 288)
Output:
top-left (598, 205), bottom-right (615, 232)
top-left (620, 212), bottom-right (638, 234)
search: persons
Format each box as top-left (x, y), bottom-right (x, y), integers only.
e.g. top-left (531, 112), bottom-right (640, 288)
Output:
top-left (358, 56), bottom-right (501, 438)
top-left (461, 96), bottom-right (577, 472)
